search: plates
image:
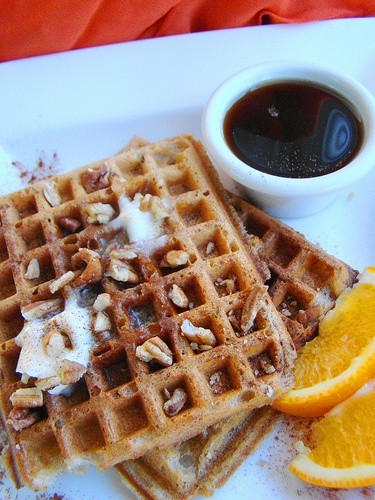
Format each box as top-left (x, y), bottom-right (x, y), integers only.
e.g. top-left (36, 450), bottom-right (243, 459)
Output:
top-left (0, 17), bottom-right (375, 500)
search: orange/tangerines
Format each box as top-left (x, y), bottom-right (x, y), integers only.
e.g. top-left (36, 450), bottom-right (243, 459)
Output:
top-left (287, 385), bottom-right (375, 488)
top-left (266, 266), bottom-right (375, 415)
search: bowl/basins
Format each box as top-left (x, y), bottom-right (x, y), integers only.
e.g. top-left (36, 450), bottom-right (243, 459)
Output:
top-left (201, 60), bottom-right (375, 219)
